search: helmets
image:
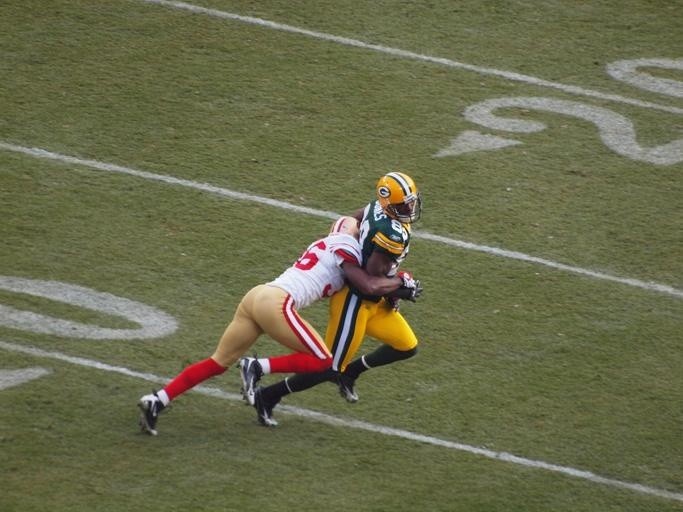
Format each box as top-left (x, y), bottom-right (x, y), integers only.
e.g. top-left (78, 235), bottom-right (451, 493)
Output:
top-left (377, 172), bottom-right (421, 221)
top-left (330, 215), bottom-right (360, 241)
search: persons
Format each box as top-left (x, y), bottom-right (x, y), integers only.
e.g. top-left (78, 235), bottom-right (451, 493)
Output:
top-left (251, 171), bottom-right (423, 430)
top-left (136, 206), bottom-right (424, 436)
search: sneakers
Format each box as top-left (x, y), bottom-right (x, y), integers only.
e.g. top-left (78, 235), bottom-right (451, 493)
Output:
top-left (239, 356), bottom-right (262, 409)
top-left (257, 386), bottom-right (278, 428)
top-left (338, 371), bottom-right (358, 403)
top-left (138, 393), bottom-right (159, 435)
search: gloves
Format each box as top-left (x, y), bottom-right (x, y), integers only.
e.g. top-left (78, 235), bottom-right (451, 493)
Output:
top-left (397, 270), bottom-right (424, 301)
